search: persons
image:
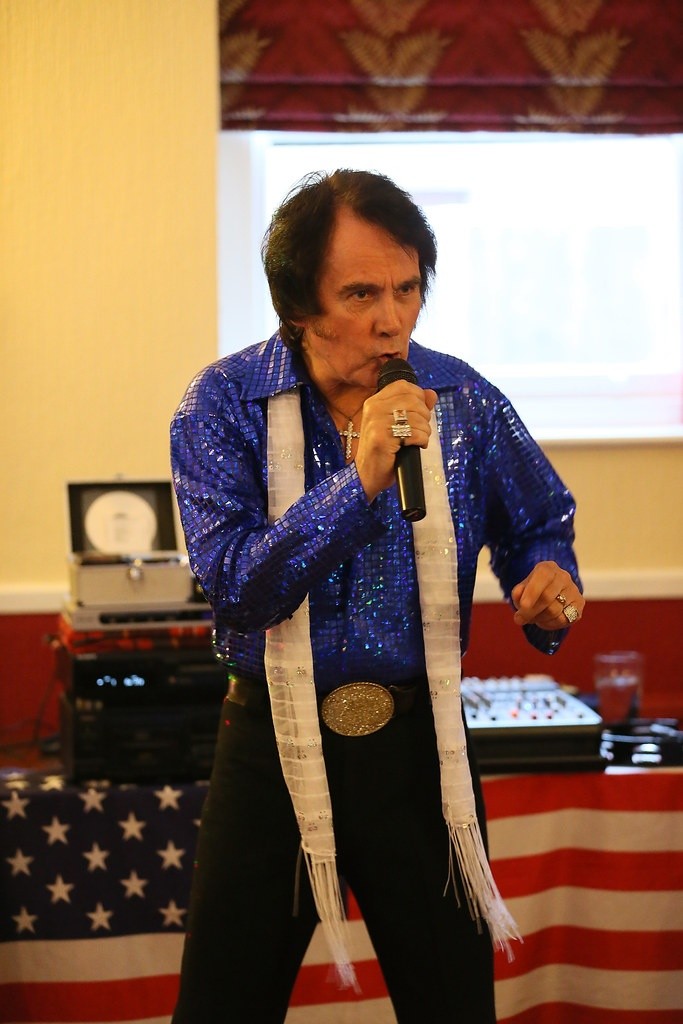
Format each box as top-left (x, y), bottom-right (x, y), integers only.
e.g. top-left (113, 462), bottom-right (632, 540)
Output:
top-left (170, 169), bottom-right (588, 1024)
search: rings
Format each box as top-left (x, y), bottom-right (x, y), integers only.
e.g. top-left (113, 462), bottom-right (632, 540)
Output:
top-left (562, 603), bottom-right (579, 623)
top-left (390, 425), bottom-right (411, 446)
top-left (391, 409), bottom-right (407, 424)
top-left (556, 594), bottom-right (567, 608)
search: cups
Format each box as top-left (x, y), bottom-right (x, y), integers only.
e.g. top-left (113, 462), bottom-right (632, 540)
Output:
top-left (593, 652), bottom-right (643, 721)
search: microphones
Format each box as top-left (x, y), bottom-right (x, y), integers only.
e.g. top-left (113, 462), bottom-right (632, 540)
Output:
top-left (376, 358), bottom-right (427, 521)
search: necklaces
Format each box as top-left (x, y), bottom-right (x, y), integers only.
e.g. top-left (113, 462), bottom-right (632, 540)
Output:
top-left (328, 391), bottom-right (377, 461)
